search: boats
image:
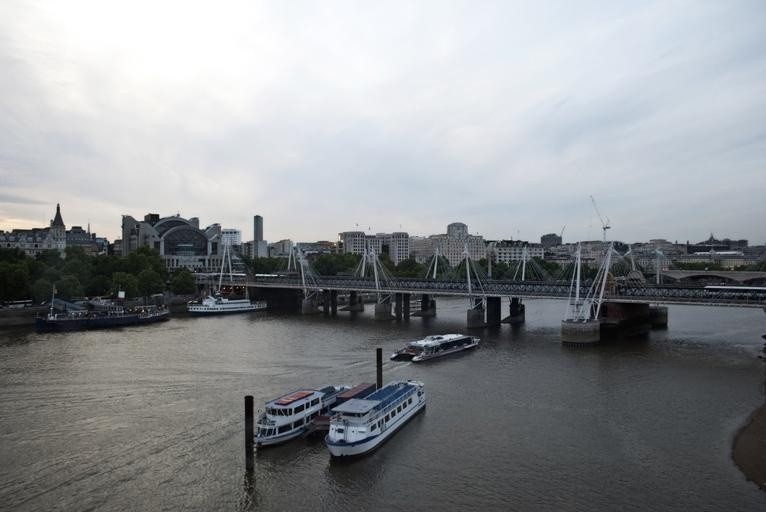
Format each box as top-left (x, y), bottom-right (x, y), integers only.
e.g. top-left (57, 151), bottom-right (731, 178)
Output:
top-left (389, 333), bottom-right (481, 362)
top-left (186, 291), bottom-right (268, 316)
top-left (253, 384), bottom-right (355, 447)
top-left (324, 379), bottom-right (426, 462)
top-left (34, 283), bottom-right (169, 333)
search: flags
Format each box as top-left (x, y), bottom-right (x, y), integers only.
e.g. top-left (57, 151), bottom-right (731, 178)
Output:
top-left (54, 285), bottom-right (58, 294)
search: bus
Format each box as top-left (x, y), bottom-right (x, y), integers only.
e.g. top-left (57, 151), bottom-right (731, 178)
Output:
top-left (189, 272), bottom-right (288, 285)
top-left (2, 299), bottom-right (31, 309)
top-left (703, 285), bottom-right (766, 300)
